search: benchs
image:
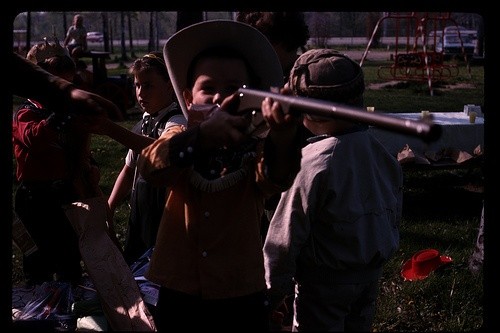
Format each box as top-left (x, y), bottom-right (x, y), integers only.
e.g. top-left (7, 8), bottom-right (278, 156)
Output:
top-left (76, 51), bottom-right (110, 84)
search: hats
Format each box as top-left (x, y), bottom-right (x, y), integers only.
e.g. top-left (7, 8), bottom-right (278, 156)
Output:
top-left (163, 19), bottom-right (285, 140)
top-left (401, 249), bottom-right (453, 281)
top-left (288, 48), bottom-right (364, 103)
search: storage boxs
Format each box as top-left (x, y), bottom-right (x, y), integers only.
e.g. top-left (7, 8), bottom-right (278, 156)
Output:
top-left (426, 173), bottom-right (461, 207)
top-left (464, 105), bottom-right (481, 117)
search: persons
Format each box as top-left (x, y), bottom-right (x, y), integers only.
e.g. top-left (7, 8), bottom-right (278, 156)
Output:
top-left (136, 19), bottom-right (301, 333)
top-left (13, 42), bottom-right (103, 286)
top-left (262, 49), bottom-right (403, 333)
top-left (107, 50), bottom-right (188, 267)
top-left (64, 15), bottom-right (88, 61)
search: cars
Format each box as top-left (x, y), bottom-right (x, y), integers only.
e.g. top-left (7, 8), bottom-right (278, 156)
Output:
top-left (87, 32), bottom-right (104, 42)
top-left (440, 26), bottom-right (472, 49)
top-left (434, 34), bottom-right (475, 60)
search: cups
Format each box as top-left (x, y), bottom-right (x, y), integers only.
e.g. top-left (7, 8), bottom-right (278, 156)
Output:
top-left (421, 110), bottom-right (430, 120)
top-left (470, 112), bottom-right (476, 123)
top-left (367, 106), bottom-right (375, 113)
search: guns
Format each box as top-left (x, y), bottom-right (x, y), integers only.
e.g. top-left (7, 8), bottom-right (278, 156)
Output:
top-left (187, 88), bottom-right (442, 143)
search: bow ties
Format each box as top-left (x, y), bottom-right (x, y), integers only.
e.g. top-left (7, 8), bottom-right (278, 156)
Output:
top-left (142, 112), bottom-right (159, 135)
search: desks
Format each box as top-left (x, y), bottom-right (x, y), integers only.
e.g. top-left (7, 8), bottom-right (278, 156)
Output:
top-left (371, 112), bottom-right (484, 175)
